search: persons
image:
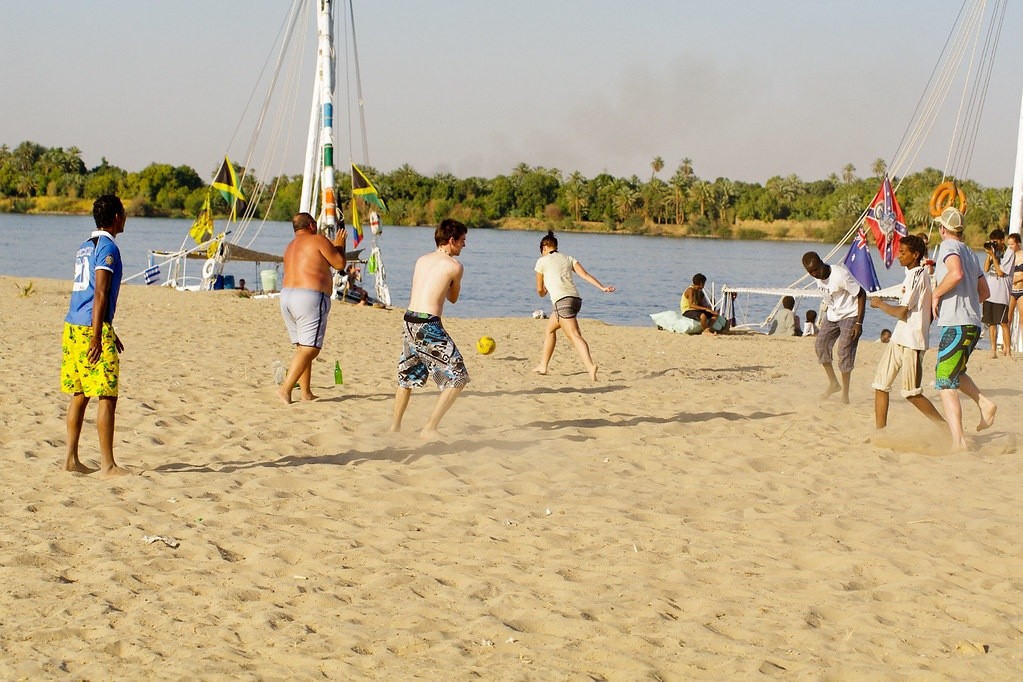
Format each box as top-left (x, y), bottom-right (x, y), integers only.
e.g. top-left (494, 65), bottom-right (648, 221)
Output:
top-left (874, 328), bottom-right (892, 343)
top-left (769, 295), bottom-right (820, 337)
top-left (277, 213), bottom-right (348, 405)
top-left (801, 251), bottom-right (866, 404)
top-left (929, 207), bottom-right (997, 452)
top-left (905, 233), bottom-right (935, 274)
top-left (981, 230), bottom-right (1016, 357)
top-left (533, 229), bottom-right (616, 381)
top-left (60, 194), bottom-right (131, 476)
top-left (680, 273), bottom-right (719, 337)
top-left (236, 278), bottom-right (248, 290)
top-left (391, 218), bottom-right (470, 439)
top-left (1006, 233), bottom-right (1023, 329)
top-left (342, 264), bottom-right (369, 304)
top-left (870, 235), bottom-right (950, 434)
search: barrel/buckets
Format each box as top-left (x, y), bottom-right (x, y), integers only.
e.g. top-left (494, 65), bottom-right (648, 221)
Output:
top-left (225, 275), bottom-right (234, 289)
top-left (214, 275), bottom-right (224, 290)
top-left (260, 269), bottom-right (277, 291)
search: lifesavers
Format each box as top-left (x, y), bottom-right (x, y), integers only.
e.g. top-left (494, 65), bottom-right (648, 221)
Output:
top-left (202, 258), bottom-right (218, 279)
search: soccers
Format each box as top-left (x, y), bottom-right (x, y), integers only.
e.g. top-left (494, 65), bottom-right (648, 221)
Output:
top-left (477, 335), bottom-right (496, 355)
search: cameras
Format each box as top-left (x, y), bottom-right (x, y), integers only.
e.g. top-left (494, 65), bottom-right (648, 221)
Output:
top-left (984, 241), bottom-right (998, 250)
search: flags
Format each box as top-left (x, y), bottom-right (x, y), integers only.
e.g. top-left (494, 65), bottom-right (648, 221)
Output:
top-left (213, 156), bottom-right (248, 221)
top-left (352, 163), bottom-right (389, 214)
top-left (353, 198), bottom-right (364, 249)
top-left (190, 191), bottom-right (215, 245)
top-left (843, 229), bottom-right (881, 294)
top-left (867, 175), bottom-right (908, 268)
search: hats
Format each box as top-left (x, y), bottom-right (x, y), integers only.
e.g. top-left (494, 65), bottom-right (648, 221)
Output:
top-left (935, 206), bottom-right (966, 233)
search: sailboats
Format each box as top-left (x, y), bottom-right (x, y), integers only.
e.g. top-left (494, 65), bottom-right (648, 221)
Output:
top-left (701, 1), bottom-right (1023, 348)
top-left (142, 0), bottom-right (391, 308)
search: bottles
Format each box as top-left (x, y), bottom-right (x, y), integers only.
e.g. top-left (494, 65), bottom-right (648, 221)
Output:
top-left (334, 361), bottom-right (343, 384)
